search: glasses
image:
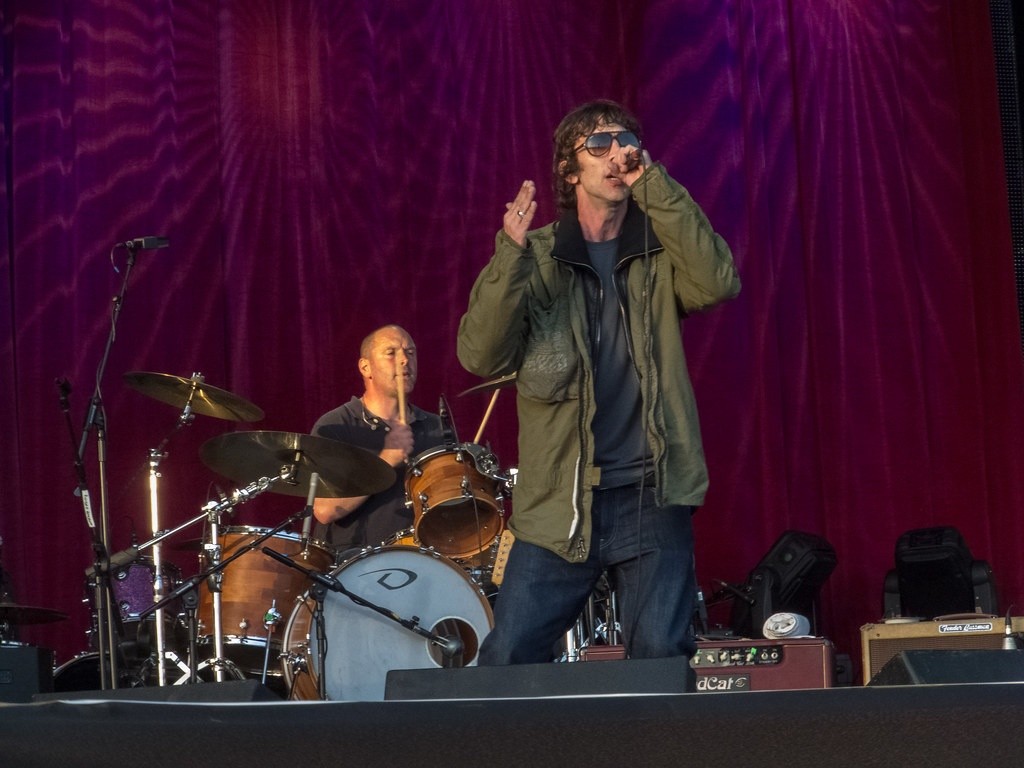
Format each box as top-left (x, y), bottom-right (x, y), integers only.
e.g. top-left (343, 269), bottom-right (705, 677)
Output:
top-left (565, 130), bottom-right (642, 159)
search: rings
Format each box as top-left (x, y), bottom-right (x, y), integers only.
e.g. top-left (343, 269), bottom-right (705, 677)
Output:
top-left (518, 211), bottom-right (524, 217)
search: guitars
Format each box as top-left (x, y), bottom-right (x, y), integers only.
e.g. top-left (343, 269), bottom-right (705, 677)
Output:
top-left (489, 526), bottom-right (516, 587)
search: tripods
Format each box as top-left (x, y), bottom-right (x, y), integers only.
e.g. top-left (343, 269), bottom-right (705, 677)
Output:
top-left (133, 408), bottom-right (300, 690)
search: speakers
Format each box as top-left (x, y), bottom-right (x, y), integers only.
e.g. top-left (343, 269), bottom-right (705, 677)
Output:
top-left (581, 641), bottom-right (832, 693)
top-left (861, 616), bottom-right (1024, 685)
top-left (31, 680), bottom-right (283, 703)
top-left (384, 655), bottom-right (696, 701)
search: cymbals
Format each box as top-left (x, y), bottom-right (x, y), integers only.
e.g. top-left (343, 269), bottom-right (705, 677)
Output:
top-left (119, 370), bottom-right (267, 423)
top-left (197, 427), bottom-right (400, 501)
top-left (1, 599), bottom-right (69, 629)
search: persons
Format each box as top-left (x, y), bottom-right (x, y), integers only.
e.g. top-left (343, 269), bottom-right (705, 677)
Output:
top-left (307, 325), bottom-right (445, 561)
top-left (457, 99), bottom-right (741, 667)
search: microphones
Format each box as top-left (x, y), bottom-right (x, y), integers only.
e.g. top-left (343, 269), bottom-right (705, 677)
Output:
top-left (116, 237), bottom-right (170, 251)
top-left (439, 397), bottom-right (453, 442)
top-left (717, 580), bottom-right (756, 607)
top-left (441, 636), bottom-right (465, 657)
top-left (626, 148), bottom-right (643, 171)
top-left (1001, 616), bottom-right (1017, 650)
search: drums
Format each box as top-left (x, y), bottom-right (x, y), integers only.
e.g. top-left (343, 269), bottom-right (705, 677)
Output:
top-left (51, 648), bottom-right (131, 694)
top-left (384, 521), bottom-right (475, 571)
top-left (87, 553), bottom-right (182, 646)
top-left (401, 440), bottom-right (509, 560)
top-left (192, 525), bottom-right (339, 656)
top-left (276, 541), bottom-right (496, 700)
